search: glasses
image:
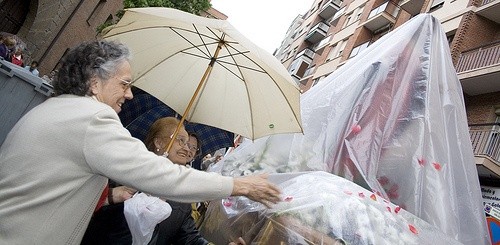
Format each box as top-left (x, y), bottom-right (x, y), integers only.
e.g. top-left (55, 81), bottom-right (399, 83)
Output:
top-left (190, 145), bottom-right (200, 152)
top-left (108, 74), bottom-right (133, 90)
top-left (162, 136), bottom-right (191, 147)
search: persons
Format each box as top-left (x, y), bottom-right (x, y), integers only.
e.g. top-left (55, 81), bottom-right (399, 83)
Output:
top-left (0, 41), bottom-right (281, 245)
top-left (0, 35), bottom-right (56, 85)
top-left (81, 117), bottom-right (246, 245)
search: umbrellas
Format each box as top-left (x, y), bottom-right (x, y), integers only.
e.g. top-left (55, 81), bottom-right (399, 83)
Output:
top-left (102, 7), bottom-right (304, 141)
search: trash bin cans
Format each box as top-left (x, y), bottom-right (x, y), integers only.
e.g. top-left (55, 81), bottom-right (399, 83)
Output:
top-left (0, 56), bottom-right (55, 151)
